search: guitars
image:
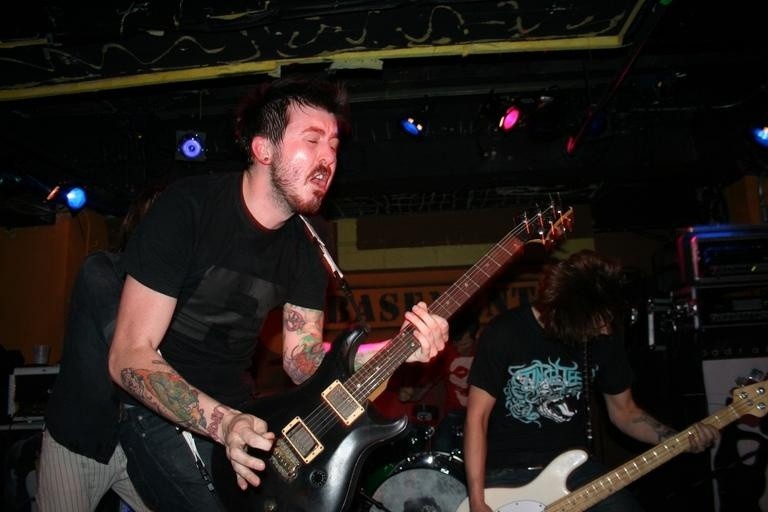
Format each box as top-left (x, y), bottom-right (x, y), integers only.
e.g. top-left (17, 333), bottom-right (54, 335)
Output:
top-left (212, 182), bottom-right (601, 511)
top-left (433, 413), bottom-right (466, 462)
top-left (452, 368), bottom-right (768, 511)
top-left (366, 422), bottom-right (427, 495)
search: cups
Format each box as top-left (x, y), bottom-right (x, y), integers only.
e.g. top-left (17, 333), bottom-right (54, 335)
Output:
top-left (32, 344), bottom-right (50, 366)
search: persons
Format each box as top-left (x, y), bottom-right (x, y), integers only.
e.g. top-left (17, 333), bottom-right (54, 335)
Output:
top-left (36, 188), bottom-right (165, 511)
top-left (462, 255), bottom-right (724, 512)
top-left (108, 70), bottom-right (451, 512)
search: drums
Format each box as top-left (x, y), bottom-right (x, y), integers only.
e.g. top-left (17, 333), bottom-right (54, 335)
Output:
top-left (366, 453), bottom-right (469, 512)
